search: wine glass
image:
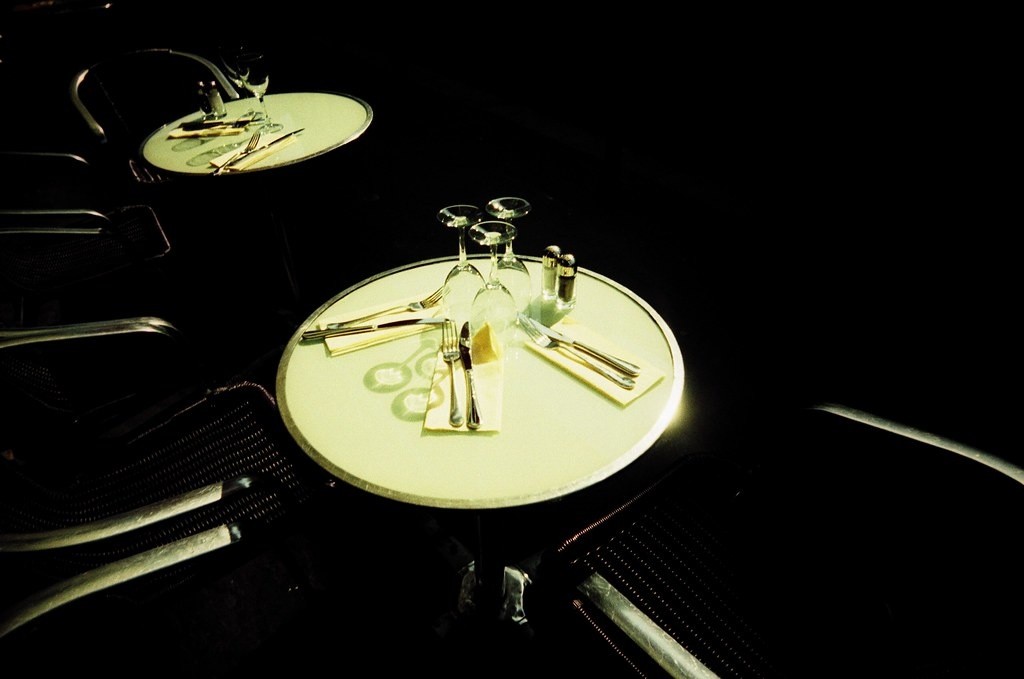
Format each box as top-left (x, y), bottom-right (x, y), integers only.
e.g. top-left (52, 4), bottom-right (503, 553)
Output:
top-left (218, 38), bottom-right (283, 133)
top-left (437, 198), bottom-right (530, 340)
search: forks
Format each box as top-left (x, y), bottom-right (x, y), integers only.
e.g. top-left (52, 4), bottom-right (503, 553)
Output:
top-left (441, 321), bottom-right (464, 427)
top-left (518, 314), bottom-right (636, 390)
top-left (328, 284), bottom-right (451, 329)
top-left (210, 134), bottom-right (260, 176)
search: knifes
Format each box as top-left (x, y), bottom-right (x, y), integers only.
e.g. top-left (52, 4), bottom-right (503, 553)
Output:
top-left (302, 317), bottom-right (449, 339)
top-left (528, 318), bottom-right (642, 376)
top-left (229, 128), bottom-right (305, 166)
top-left (459, 322), bottom-right (481, 430)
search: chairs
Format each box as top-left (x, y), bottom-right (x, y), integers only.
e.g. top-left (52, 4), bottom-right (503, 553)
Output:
top-left (0, 521), bottom-right (481, 679)
top-left (542, 396), bottom-right (1022, 679)
top-left (1, 323), bottom-right (343, 564)
top-left (1, 149), bottom-right (115, 227)
top-left (2, 223), bottom-right (178, 351)
top-left (71, 42), bottom-right (252, 152)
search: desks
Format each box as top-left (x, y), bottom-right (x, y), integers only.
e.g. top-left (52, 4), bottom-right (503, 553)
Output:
top-left (273, 247), bottom-right (696, 630)
top-left (146, 84), bottom-right (379, 188)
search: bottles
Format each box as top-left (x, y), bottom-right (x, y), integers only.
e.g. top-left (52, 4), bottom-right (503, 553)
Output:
top-left (542, 244), bottom-right (578, 308)
top-left (197, 80), bottom-right (227, 118)
